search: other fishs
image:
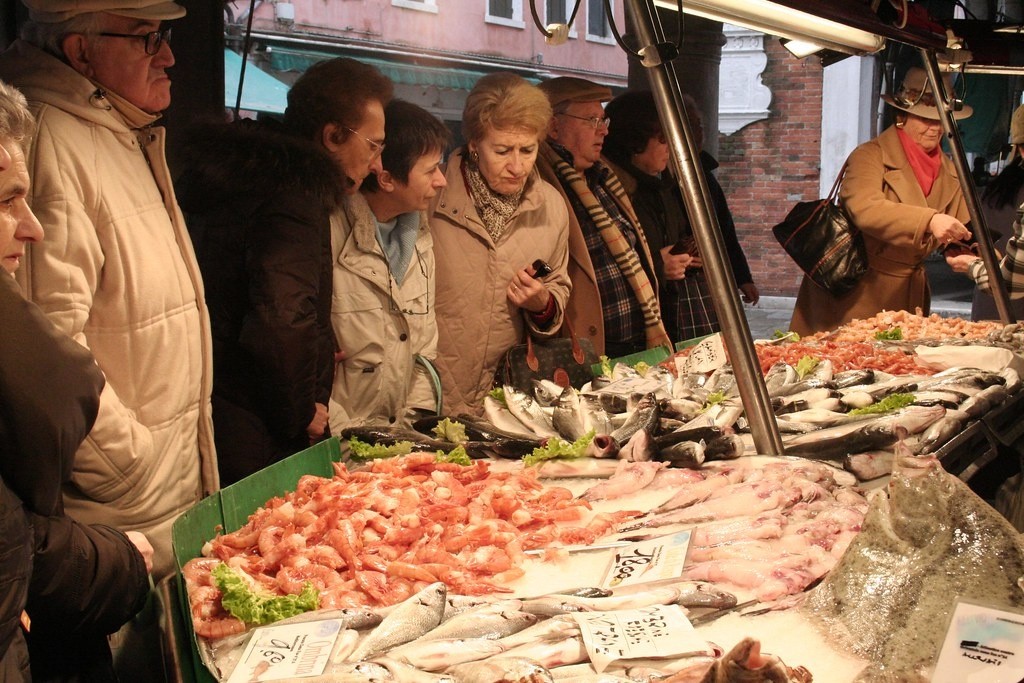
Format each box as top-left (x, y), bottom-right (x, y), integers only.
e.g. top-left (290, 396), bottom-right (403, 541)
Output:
top-left (207, 580), bottom-right (794, 683)
top-left (341, 359), bottom-right (1007, 469)
top-left (794, 441), bottom-right (1023, 683)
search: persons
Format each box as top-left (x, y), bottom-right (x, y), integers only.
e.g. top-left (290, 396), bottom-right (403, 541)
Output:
top-left (945, 103), bottom-right (1024, 300)
top-left (788, 69), bottom-right (973, 338)
top-left (0, 0), bottom-right (219, 683)
top-left (203, 56), bottom-right (759, 487)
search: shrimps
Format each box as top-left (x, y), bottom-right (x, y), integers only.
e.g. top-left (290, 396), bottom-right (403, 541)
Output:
top-left (657, 339), bottom-right (938, 379)
top-left (180, 451), bottom-right (645, 639)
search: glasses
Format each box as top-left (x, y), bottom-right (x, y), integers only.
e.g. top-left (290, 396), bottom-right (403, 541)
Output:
top-left (554, 113), bottom-right (610, 130)
top-left (649, 134), bottom-right (667, 143)
top-left (341, 124), bottom-right (386, 164)
top-left (63, 26), bottom-right (172, 54)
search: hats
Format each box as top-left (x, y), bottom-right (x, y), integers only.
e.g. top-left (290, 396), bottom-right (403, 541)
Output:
top-left (1008, 104), bottom-right (1024, 144)
top-left (535, 76), bottom-right (612, 109)
top-left (881, 68), bottom-right (973, 120)
top-left (24, 0), bottom-right (187, 24)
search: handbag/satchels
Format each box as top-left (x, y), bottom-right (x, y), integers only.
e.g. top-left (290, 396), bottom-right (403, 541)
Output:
top-left (770, 141), bottom-right (890, 298)
top-left (493, 306), bottom-right (600, 402)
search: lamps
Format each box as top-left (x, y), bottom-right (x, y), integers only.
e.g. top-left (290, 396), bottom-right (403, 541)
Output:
top-left (778, 38), bottom-right (829, 62)
top-left (653, 0), bottom-right (887, 60)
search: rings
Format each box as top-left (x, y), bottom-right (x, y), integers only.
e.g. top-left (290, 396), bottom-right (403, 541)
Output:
top-left (946, 236), bottom-right (954, 243)
top-left (512, 286), bottom-right (517, 292)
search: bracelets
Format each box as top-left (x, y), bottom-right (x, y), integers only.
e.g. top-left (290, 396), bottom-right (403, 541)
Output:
top-left (967, 257), bottom-right (983, 278)
